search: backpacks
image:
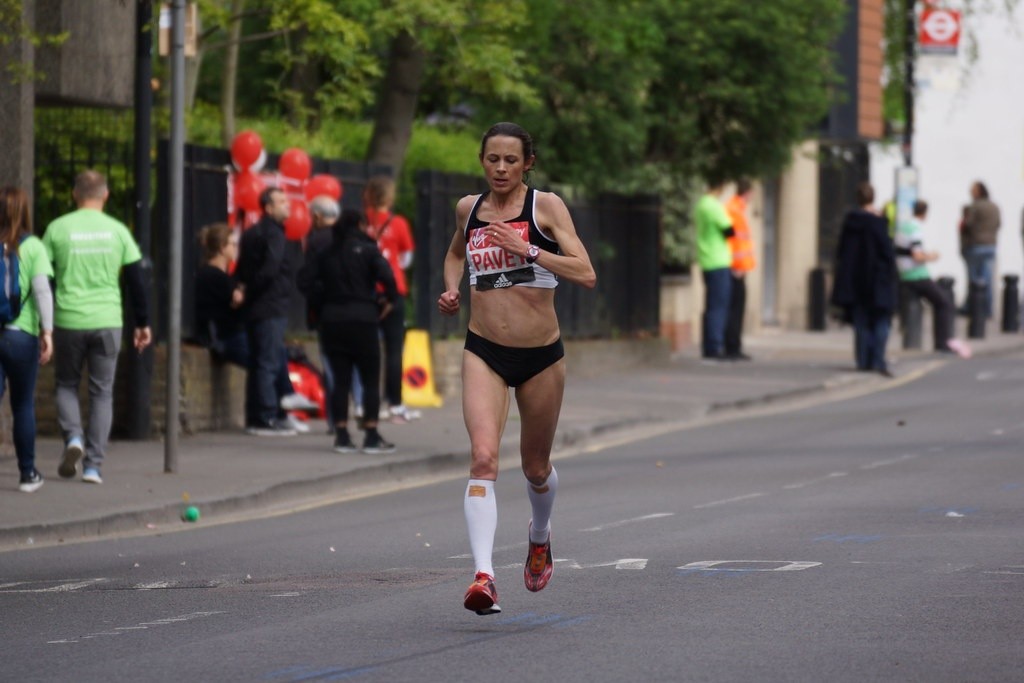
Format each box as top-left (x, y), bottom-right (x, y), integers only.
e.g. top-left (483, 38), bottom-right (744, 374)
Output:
top-left (0, 233), bottom-right (30, 324)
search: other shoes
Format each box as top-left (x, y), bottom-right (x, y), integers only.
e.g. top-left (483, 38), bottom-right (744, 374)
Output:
top-left (246, 413), bottom-right (308, 437)
top-left (353, 405), bottom-right (388, 419)
top-left (387, 404), bottom-right (419, 419)
top-left (281, 394), bottom-right (317, 410)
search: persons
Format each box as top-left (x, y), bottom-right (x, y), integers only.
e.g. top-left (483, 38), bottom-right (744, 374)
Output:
top-left (693, 166), bottom-right (756, 363)
top-left (305, 209), bottom-right (399, 454)
top-left (960, 181), bottom-right (1001, 319)
top-left (438, 121), bottom-right (597, 615)
top-left (831, 184), bottom-right (899, 380)
top-left (0, 184), bottom-right (55, 494)
top-left (41, 169), bottom-right (151, 484)
top-left (197, 177), bottom-right (421, 436)
top-left (895, 199), bottom-right (960, 354)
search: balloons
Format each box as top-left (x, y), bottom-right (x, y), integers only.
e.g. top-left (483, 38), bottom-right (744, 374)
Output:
top-left (231, 130), bottom-right (342, 240)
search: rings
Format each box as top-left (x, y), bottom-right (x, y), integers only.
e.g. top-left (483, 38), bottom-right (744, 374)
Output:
top-left (494, 231), bottom-right (498, 237)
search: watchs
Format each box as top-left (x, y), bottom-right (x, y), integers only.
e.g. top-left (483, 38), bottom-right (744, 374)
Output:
top-left (526, 245), bottom-right (540, 264)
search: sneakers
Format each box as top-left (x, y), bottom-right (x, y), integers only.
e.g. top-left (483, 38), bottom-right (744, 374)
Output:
top-left (523, 518), bottom-right (554, 591)
top-left (464, 570), bottom-right (501, 615)
top-left (58, 436), bottom-right (83, 478)
top-left (334, 428), bottom-right (356, 453)
top-left (82, 466), bottom-right (103, 484)
top-left (18, 468), bottom-right (43, 492)
top-left (362, 427), bottom-right (396, 454)
